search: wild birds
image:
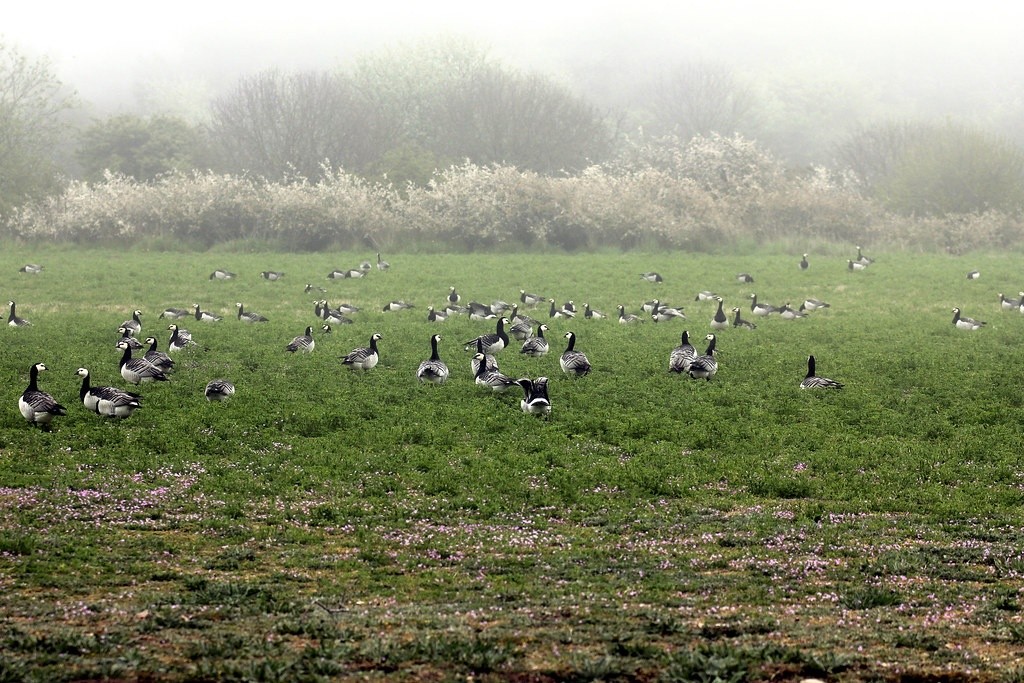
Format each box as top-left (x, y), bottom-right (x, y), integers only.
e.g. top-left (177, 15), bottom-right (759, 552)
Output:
top-left (287, 250), bottom-right (846, 417)
top-left (735, 273), bottom-right (754, 284)
top-left (5, 262), bottom-right (287, 428)
top-left (966, 270), bottom-right (981, 282)
top-left (799, 253), bottom-right (810, 269)
top-left (950, 307), bottom-right (987, 330)
top-left (845, 243), bottom-right (876, 272)
top-left (997, 290), bottom-right (1024, 316)
